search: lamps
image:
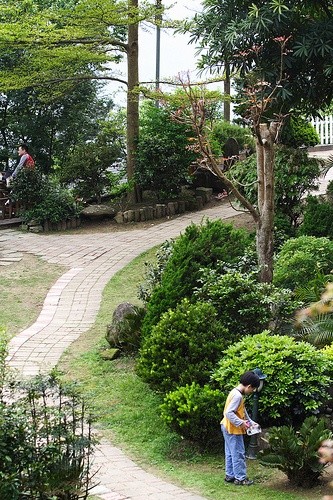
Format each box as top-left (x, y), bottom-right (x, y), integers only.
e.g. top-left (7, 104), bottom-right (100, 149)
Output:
top-left (250, 369), bottom-right (268, 393)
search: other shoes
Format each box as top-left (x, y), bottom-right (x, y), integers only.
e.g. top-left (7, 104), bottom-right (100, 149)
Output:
top-left (234, 479), bottom-right (253, 485)
top-left (225, 475), bottom-right (235, 482)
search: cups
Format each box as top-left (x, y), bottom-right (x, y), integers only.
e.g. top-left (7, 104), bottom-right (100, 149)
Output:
top-left (246, 425), bottom-right (261, 435)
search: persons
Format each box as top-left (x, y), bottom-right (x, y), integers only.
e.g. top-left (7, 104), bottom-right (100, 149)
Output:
top-left (220, 372), bottom-right (260, 485)
top-left (4, 144), bottom-right (35, 206)
